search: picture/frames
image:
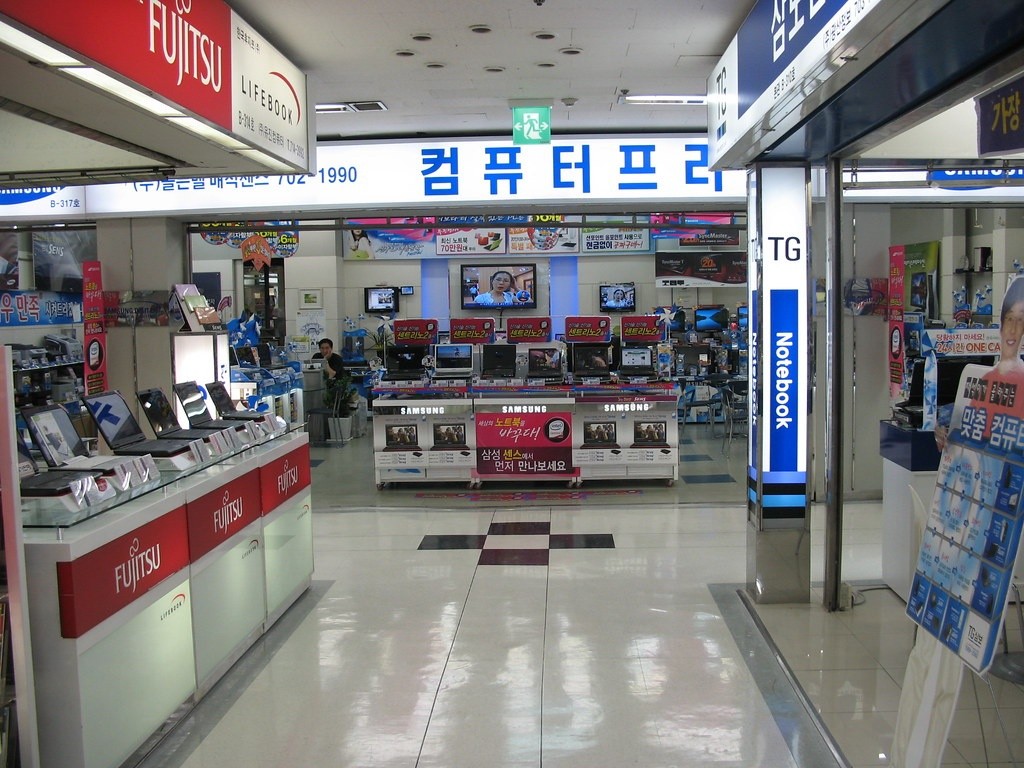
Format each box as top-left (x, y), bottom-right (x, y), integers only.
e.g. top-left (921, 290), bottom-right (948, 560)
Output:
top-left (299, 287), bottom-right (323, 309)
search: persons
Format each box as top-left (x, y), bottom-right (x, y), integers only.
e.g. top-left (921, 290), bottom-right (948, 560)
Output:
top-left (436, 426), bottom-right (463, 441)
top-left (636, 423), bottom-right (664, 440)
top-left (312, 338), bottom-right (344, 378)
top-left (388, 428), bottom-right (416, 442)
top-left (351, 229), bottom-right (370, 258)
top-left (470, 271), bottom-right (534, 302)
top-left (970, 278), bottom-right (1024, 438)
top-left (587, 424), bottom-right (614, 441)
top-left (606, 289), bottom-right (628, 306)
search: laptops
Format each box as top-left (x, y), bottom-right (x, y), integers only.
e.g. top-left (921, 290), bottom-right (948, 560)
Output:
top-left (894, 358), bottom-right (982, 407)
top-left (16, 343), bottom-right (291, 497)
top-left (383, 424), bottom-right (422, 451)
top-left (475, 232), bottom-right (502, 250)
top-left (580, 421), bottom-right (621, 449)
top-left (380, 341), bottom-right (658, 382)
top-left (630, 421), bottom-right (670, 448)
top-left (430, 423), bottom-right (471, 450)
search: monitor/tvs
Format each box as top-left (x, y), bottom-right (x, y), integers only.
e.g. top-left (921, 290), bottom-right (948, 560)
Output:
top-left (694, 308), bottom-right (727, 331)
top-left (738, 306), bottom-right (749, 331)
top-left (600, 286), bottom-right (636, 312)
top-left (673, 343), bottom-right (711, 372)
top-left (461, 264), bottom-right (537, 310)
top-left (654, 309), bottom-right (685, 332)
top-left (364, 287), bottom-right (399, 313)
top-left (910, 272), bottom-right (927, 312)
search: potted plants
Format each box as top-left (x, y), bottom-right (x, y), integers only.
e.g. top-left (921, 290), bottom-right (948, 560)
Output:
top-left (324, 371), bottom-right (353, 444)
top-left (364, 327), bottom-right (395, 366)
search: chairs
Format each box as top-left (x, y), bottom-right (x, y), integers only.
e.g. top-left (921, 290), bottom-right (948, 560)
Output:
top-left (306, 385), bottom-right (345, 450)
top-left (680, 372), bottom-right (748, 461)
top-left (990, 584), bottom-right (1024, 687)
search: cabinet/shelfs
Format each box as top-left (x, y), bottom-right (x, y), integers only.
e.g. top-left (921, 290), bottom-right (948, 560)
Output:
top-left (0, 324), bottom-right (678, 767)
top-left (845, 262), bottom-right (1024, 607)
